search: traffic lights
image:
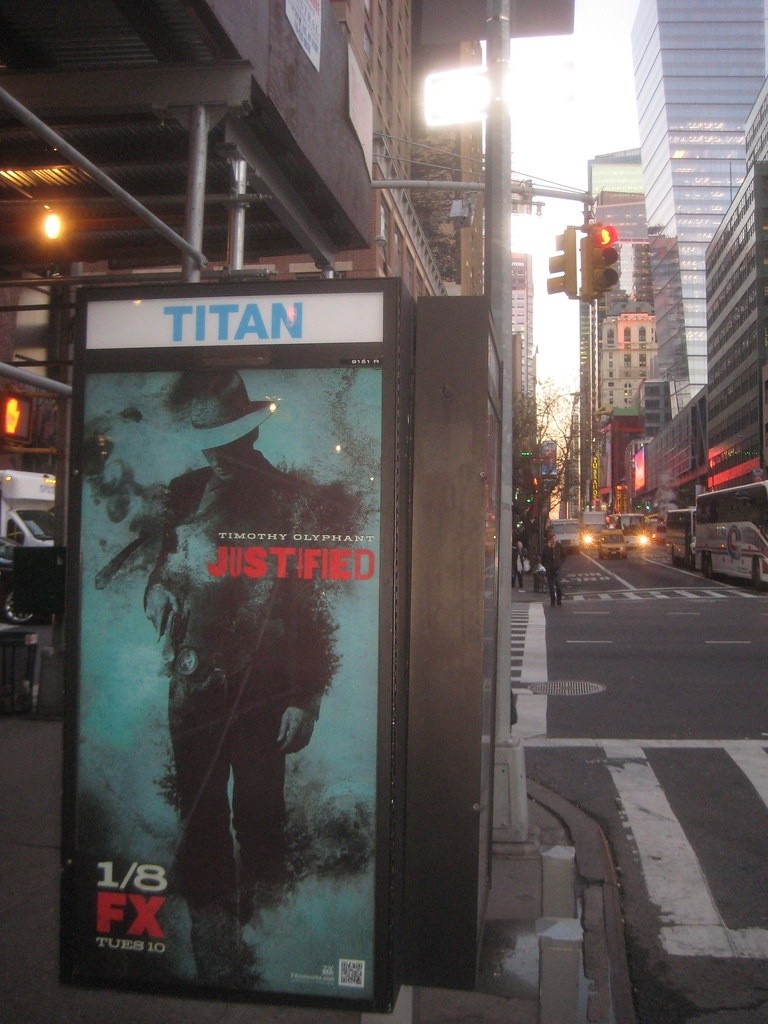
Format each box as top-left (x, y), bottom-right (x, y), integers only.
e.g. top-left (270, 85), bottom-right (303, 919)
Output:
top-left (2, 391), bottom-right (35, 446)
top-left (585, 222), bottom-right (619, 293)
top-left (547, 233), bottom-right (579, 298)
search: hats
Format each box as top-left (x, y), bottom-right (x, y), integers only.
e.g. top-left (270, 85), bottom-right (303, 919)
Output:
top-left (177, 370), bottom-right (279, 450)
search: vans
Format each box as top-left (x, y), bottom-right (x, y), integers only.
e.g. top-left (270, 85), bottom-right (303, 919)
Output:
top-left (600, 527), bottom-right (631, 562)
top-left (549, 518), bottom-right (582, 556)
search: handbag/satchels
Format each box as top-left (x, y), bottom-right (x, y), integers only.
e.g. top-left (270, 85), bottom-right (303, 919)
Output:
top-left (523, 559), bottom-right (530, 572)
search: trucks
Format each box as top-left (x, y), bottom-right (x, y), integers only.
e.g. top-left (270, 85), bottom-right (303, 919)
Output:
top-left (580, 510), bottom-right (608, 551)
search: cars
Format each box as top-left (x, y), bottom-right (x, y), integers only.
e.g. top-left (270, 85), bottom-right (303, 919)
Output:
top-left (0, 537), bottom-right (47, 627)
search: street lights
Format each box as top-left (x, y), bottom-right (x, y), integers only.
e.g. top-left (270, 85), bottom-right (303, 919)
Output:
top-left (538, 391), bottom-right (580, 476)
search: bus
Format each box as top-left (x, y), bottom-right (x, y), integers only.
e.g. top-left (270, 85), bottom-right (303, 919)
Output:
top-left (696, 480), bottom-right (768, 592)
top-left (611, 511), bottom-right (652, 551)
top-left (0, 468), bottom-right (57, 554)
top-left (666, 509), bottom-right (696, 572)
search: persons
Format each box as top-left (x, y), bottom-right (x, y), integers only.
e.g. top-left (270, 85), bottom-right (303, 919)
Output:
top-left (139, 370), bottom-right (332, 992)
top-left (540, 533), bottom-right (566, 608)
top-left (513, 541), bottom-right (528, 592)
